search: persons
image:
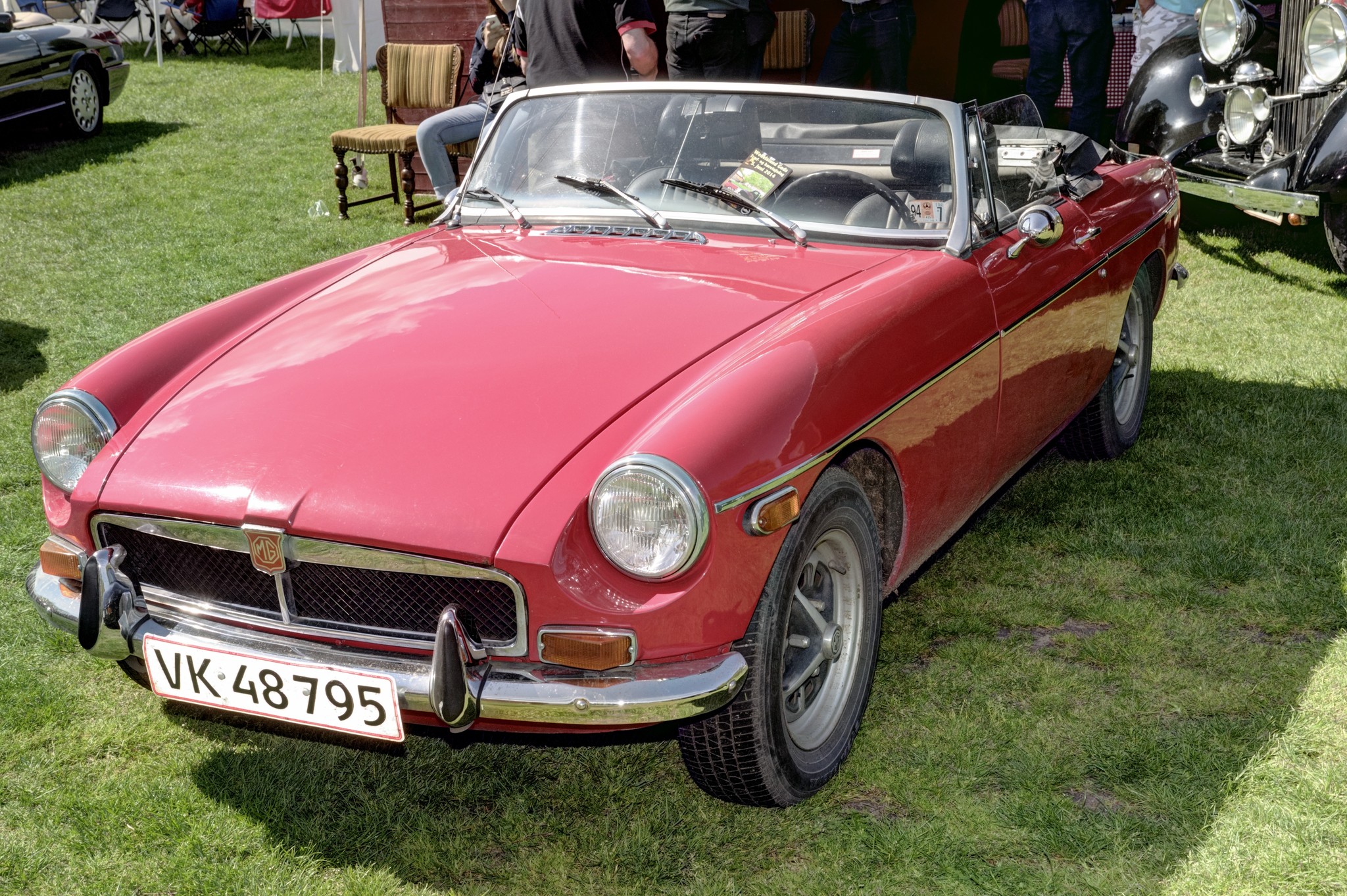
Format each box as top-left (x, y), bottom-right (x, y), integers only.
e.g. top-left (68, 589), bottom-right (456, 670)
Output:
top-left (815, 0), bottom-right (916, 94)
top-left (1129, 0), bottom-right (1207, 85)
top-left (1016, 0), bottom-right (1114, 142)
top-left (416, 0), bottom-right (759, 208)
top-left (163, 0), bottom-right (203, 54)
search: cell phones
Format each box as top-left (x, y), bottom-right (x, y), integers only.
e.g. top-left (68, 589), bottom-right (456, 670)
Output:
top-left (486, 15), bottom-right (509, 35)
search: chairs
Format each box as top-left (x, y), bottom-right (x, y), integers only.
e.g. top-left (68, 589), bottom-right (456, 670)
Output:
top-left (330, 42), bottom-right (526, 225)
top-left (761, 9), bottom-right (813, 84)
top-left (15, 0), bottom-right (251, 59)
top-left (248, 0), bottom-right (332, 53)
top-left (994, 0), bottom-right (1033, 83)
top-left (622, 93), bottom-right (769, 216)
top-left (843, 114), bottom-right (1011, 232)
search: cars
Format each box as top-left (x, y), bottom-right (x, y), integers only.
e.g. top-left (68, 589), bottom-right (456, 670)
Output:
top-left (0, 8), bottom-right (131, 154)
top-left (22, 73), bottom-right (1182, 808)
top-left (1115, 0), bottom-right (1346, 286)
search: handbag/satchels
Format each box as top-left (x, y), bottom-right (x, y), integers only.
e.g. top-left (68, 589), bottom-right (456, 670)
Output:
top-left (482, 75), bottom-right (527, 108)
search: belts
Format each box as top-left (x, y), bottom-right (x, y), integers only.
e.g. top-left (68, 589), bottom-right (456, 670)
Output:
top-left (848, 0), bottom-right (897, 15)
